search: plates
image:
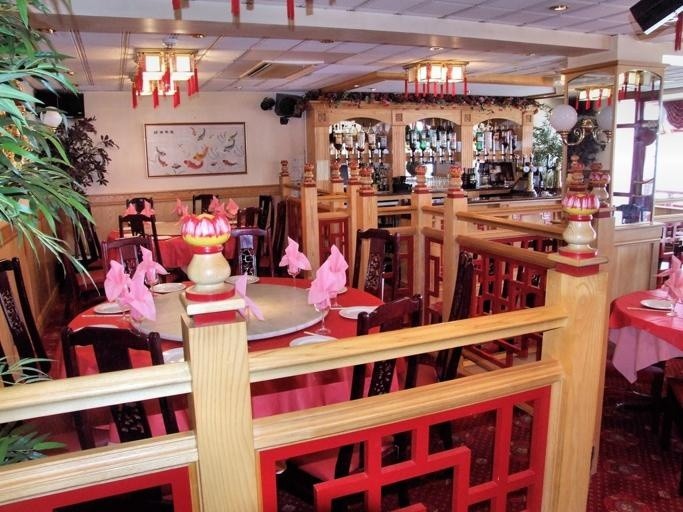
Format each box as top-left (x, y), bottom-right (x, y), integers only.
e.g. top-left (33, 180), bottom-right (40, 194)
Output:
top-left (160, 346), bottom-right (186, 364)
top-left (286, 336), bottom-right (339, 346)
top-left (223, 273), bottom-right (260, 285)
top-left (639, 297), bottom-right (673, 310)
top-left (335, 304), bottom-right (378, 320)
top-left (118, 225), bottom-right (172, 240)
top-left (150, 281), bottom-right (186, 293)
top-left (92, 301), bottom-right (133, 314)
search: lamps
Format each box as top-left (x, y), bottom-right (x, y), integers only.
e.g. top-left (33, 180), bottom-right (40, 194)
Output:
top-left (549, 105), bottom-right (589, 147)
top-left (131, 47), bottom-right (200, 111)
top-left (574, 85), bottom-right (612, 110)
top-left (400, 59), bottom-right (470, 99)
top-left (590, 104), bottom-right (610, 149)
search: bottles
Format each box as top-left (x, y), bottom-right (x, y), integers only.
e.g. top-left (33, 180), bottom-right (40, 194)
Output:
top-left (532, 158), bottom-right (563, 195)
top-left (462, 168), bottom-right (476, 189)
top-left (329, 121), bottom-right (388, 150)
top-left (404, 119), bottom-right (458, 149)
top-left (479, 164), bottom-right (514, 188)
top-left (372, 167), bottom-right (389, 192)
top-left (473, 122), bottom-right (514, 143)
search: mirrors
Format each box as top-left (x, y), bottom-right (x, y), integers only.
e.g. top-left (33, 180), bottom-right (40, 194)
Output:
top-left (610, 68), bottom-right (664, 226)
top-left (563, 66), bottom-right (613, 209)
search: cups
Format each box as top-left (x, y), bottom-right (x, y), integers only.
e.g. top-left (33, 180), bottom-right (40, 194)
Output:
top-left (407, 175), bottom-right (449, 190)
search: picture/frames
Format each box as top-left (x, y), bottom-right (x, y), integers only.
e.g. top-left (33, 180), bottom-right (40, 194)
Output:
top-left (143, 122), bottom-right (247, 177)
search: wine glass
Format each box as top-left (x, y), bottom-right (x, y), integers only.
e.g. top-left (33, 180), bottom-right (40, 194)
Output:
top-left (117, 269), bottom-right (158, 334)
top-left (286, 257), bottom-right (302, 286)
top-left (309, 294), bottom-right (334, 335)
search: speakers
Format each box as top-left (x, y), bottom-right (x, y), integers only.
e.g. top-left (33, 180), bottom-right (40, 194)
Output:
top-left (32, 87), bottom-right (86, 120)
top-left (275, 93), bottom-right (303, 118)
top-left (627, 0), bottom-right (683, 37)
